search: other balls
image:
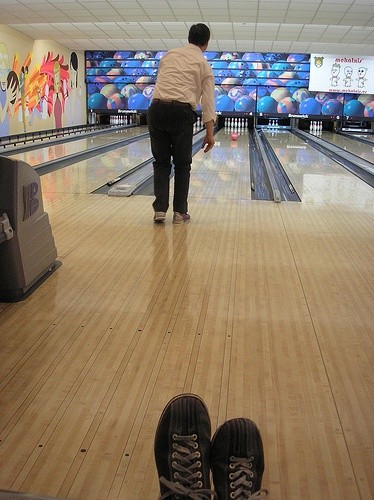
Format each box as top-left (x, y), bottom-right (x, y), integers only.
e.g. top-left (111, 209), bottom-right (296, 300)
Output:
top-left (231, 133), bottom-right (238, 140)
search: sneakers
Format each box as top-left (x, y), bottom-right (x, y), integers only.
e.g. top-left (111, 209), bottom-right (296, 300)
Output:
top-left (154, 211), bottom-right (166, 222)
top-left (209, 418), bottom-right (268, 500)
top-left (154, 392), bottom-right (211, 500)
top-left (173, 212), bottom-right (190, 224)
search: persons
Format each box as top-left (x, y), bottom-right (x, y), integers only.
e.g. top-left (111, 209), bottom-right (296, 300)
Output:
top-left (146, 23), bottom-right (216, 224)
top-left (154, 394), bottom-right (270, 500)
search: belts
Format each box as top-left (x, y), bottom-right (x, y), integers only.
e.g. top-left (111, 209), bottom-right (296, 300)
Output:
top-left (152, 99), bottom-right (189, 107)
